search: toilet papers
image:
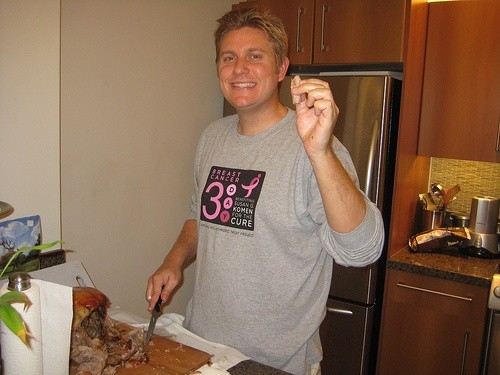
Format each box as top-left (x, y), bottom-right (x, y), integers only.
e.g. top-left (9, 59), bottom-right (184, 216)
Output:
top-left (0, 278), bottom-right (74, 375)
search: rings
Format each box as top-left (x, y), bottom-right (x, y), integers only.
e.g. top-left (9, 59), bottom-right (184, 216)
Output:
top-left (147, 296), bottom-right (152, 300)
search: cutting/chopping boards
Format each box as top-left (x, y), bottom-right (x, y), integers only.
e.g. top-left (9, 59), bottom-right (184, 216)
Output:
top-left (115, 321), bottom-right (211, 375)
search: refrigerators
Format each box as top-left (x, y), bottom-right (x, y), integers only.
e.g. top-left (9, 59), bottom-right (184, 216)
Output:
top-left (223, 62), bottom-right (403, 375)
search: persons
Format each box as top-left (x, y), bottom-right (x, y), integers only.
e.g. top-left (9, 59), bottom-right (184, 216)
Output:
top-left (145, 7), bottom-right (385, 375)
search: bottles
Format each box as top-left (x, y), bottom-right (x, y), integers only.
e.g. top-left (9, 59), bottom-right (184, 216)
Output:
top-left (0, 273), bottom-right (44, 375)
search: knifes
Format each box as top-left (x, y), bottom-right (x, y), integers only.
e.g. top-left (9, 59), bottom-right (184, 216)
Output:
top-left (146, 284), bottom-right (166, 344)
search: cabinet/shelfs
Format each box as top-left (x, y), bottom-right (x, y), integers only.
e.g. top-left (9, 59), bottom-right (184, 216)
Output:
top-left (224, 0), bottom-right (500, 375)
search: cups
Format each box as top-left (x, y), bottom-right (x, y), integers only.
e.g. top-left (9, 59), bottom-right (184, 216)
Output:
top-left (422, 209), bottom-right (442, 231)
